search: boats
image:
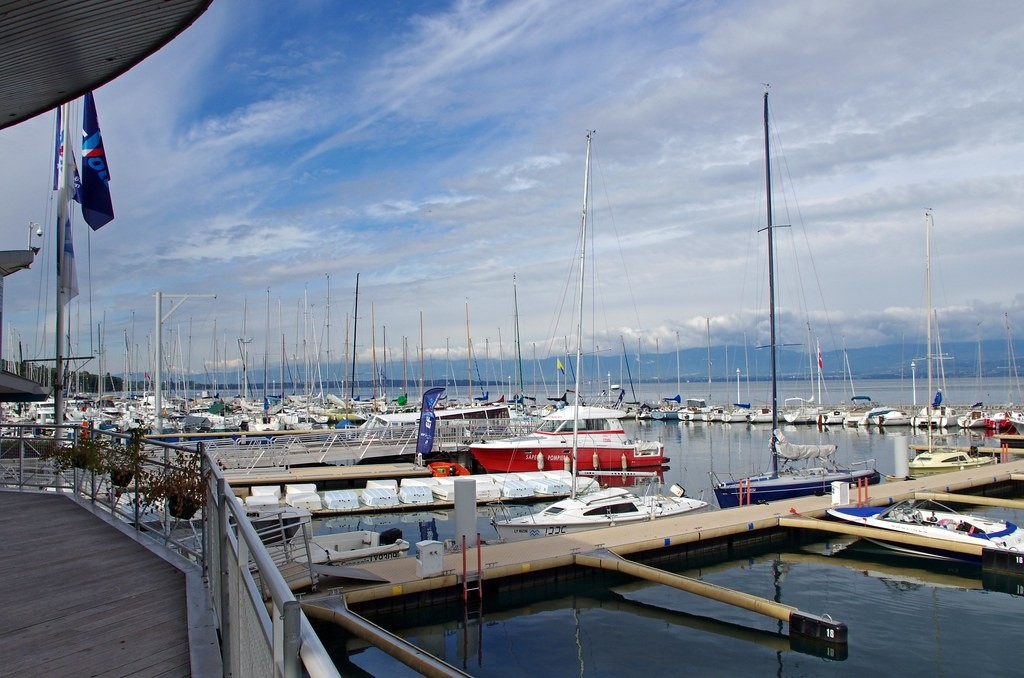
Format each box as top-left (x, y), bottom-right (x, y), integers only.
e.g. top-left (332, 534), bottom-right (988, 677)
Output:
top-left (261, 530), bottom-right (409, 569)
top-left (825, 507), bottom-right (1024, 581)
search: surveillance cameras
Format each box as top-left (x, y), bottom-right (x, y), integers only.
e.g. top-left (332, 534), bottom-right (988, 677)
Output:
top-left (37, 229), bottom-right (43, 237)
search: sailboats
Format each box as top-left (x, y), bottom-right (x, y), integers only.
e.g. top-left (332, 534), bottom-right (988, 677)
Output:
top-left (0, 211), bottom-right (1024, 446)
top-left (490, 130), bottom-right (709, 540)
top-left (705, 81), bottom-right (880, 508)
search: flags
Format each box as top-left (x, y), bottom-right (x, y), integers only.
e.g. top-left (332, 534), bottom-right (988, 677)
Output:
top-left (818, 340), bottom-right (824, 369)
top-left (558, 358), bottom-right (565, 375)
top-left (144, 371), bottom-right (150, 382)
top-left (53, 105), bottom-right (81, 204)
top-left (81, 91), bottom-right (115, 231)
top-left (56, 105), bottom-right (79, 307)
